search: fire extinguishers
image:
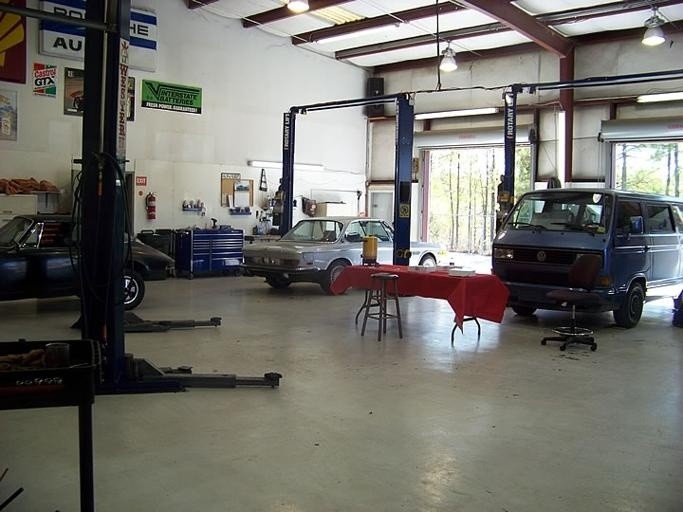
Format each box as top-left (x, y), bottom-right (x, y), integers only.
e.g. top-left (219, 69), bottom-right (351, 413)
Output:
top-left (146, 192), bottom-right (157, 219)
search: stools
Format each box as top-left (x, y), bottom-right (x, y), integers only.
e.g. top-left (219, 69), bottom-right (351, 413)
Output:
top-left (360, 272), bottom-right (404, 343)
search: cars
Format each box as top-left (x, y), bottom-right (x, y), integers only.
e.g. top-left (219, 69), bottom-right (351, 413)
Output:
top-left (239, 216), bottom-right (439, 296)
top-left (72, 93), bottom-right (84, 111)
top-left (236, 183), bottom-right (249, 191)
top-left (0, 213), bottom-right (177, 311)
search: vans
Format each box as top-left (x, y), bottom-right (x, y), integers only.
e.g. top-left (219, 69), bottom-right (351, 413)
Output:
top-left (491, 188), bottom-right (683, 328)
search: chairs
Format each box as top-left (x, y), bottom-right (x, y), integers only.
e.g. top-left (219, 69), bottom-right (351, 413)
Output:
top-left (542, 253), bottom-right (603, 354)
top-left (322, 229), bottom-right (336, 241)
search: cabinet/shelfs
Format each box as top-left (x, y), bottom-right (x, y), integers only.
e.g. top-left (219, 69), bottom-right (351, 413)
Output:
top-left (0, 193), bottom-right (38, 244)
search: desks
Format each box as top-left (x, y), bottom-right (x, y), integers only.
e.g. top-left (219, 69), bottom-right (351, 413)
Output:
top-left (344, 261), bottom-right (496, 344)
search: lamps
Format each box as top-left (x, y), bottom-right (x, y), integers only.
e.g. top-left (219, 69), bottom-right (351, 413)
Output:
top-left (640, 2), bottom-right (668, 47)
top-left (439, 39), bottom-right (459, 74)
